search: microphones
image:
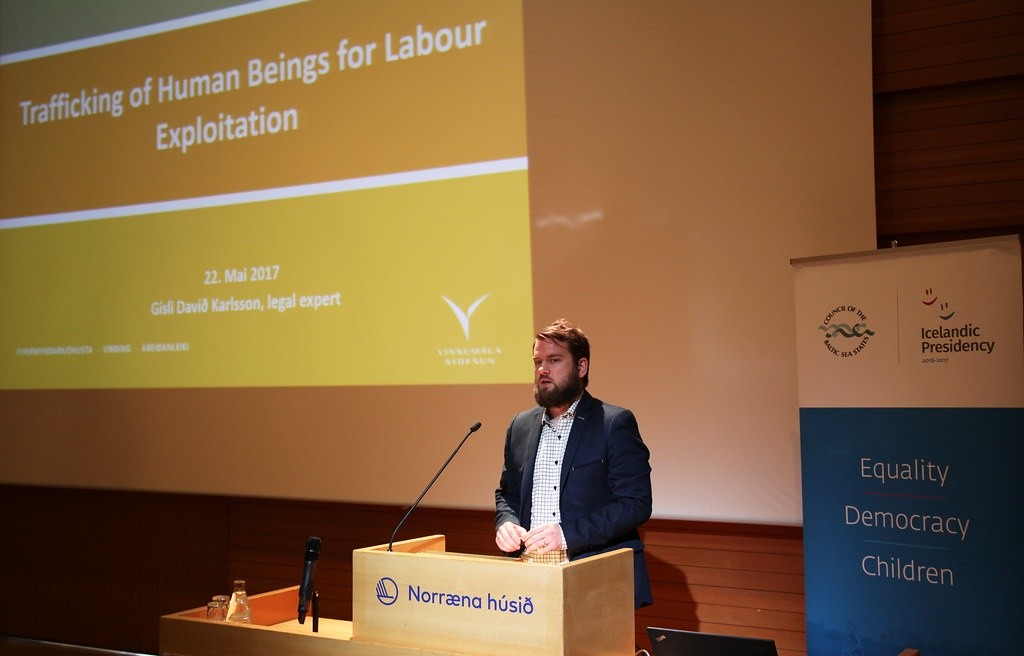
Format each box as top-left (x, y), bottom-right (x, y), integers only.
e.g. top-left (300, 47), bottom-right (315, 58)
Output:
top-left (386, 421), bottom-right (483, 552)
top-left (297, 536), bottom-right (322, 625)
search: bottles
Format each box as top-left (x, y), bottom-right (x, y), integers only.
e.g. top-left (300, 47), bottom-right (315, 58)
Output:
top-left (226, 579), bottom-right (252, 623)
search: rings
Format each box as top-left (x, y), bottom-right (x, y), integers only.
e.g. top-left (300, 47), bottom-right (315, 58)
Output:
top-left (541, 540), bottom-right (544, 547)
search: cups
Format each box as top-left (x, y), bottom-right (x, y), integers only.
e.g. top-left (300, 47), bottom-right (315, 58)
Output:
top-left (207, 602), bottom-right (223, 620)
top-left (213, 595), bottom-right (230, 618)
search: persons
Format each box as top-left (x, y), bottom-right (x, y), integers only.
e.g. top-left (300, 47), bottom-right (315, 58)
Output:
top-left (494, 320), bottom-right (654, 610)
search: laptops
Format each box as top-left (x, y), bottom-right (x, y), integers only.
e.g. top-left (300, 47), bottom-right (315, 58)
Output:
top-left (646, 627), bottom-right (779, 656)
